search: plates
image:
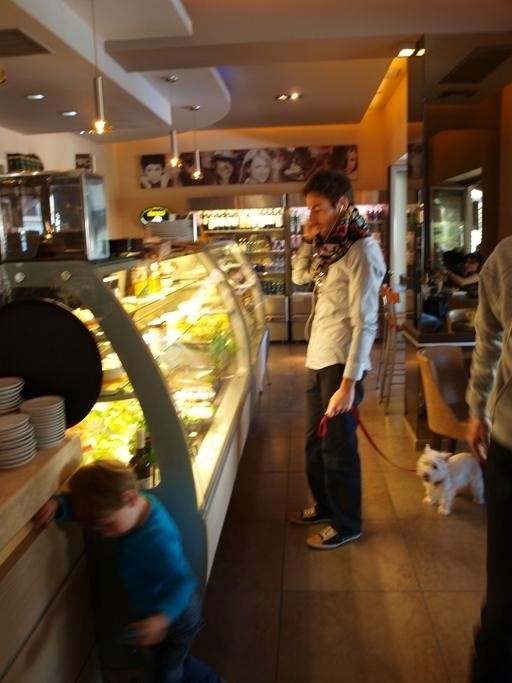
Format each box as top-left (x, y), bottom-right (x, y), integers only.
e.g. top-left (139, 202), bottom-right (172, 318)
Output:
top-left (0, 436), bottom-right (109, 683)
top-left (0, 168), bottom-right (110, 262)
top-left (0, 241), bottom-right (271, 612)
top-left (188, 194), bottom-right (289, 344)
top-left (289, 190), bottom-right (389, 341)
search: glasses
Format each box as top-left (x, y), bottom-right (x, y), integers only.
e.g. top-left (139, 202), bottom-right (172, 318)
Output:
top-left (84, 1), bottom-right (113, 140)
top-left (162, 75), bottom-right (183, 170)
top-left (190, 105), bottom-right (204, 183)
top-left (392, 39), bottom-right (416, 58)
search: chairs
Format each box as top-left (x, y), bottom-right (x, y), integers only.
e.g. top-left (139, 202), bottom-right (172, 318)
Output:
top-left (288, 505), bottom-right (330, 524)
top-left (306, 525), bottom-right (360, 549)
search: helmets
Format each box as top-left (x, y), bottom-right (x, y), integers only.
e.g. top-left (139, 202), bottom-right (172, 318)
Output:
top-left (0, 377), bottom-right (26, 415)
top-left (20, 396), bottom-right (66, 446)
top-left (1, 412), bottom-right (35, 468)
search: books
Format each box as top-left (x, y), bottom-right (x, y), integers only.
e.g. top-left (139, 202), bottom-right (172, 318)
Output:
top-left (144, 212), bottom-right (197, 243)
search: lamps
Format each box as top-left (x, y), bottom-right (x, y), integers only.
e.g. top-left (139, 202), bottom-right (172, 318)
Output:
top-left (372, 250), bottom-right (512, 457)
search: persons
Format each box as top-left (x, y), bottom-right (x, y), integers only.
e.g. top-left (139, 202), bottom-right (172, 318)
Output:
top-left (137, 144), bottom-right (361, 191)
top-left (291, 170), bottom-right (385, 550)
top-left (31, 458), bottom-right (221, 681)
top-left (415, 240), bottom-right (485, 333)
top-left (460, 232), bottom-right (511, 682)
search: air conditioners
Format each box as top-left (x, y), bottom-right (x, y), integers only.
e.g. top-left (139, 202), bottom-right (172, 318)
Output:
top-left (415, 442), bottom-right (486, 515)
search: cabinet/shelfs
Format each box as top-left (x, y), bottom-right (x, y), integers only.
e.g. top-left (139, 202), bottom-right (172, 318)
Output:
top-left (198, 207), bottom-right (284, 232)
